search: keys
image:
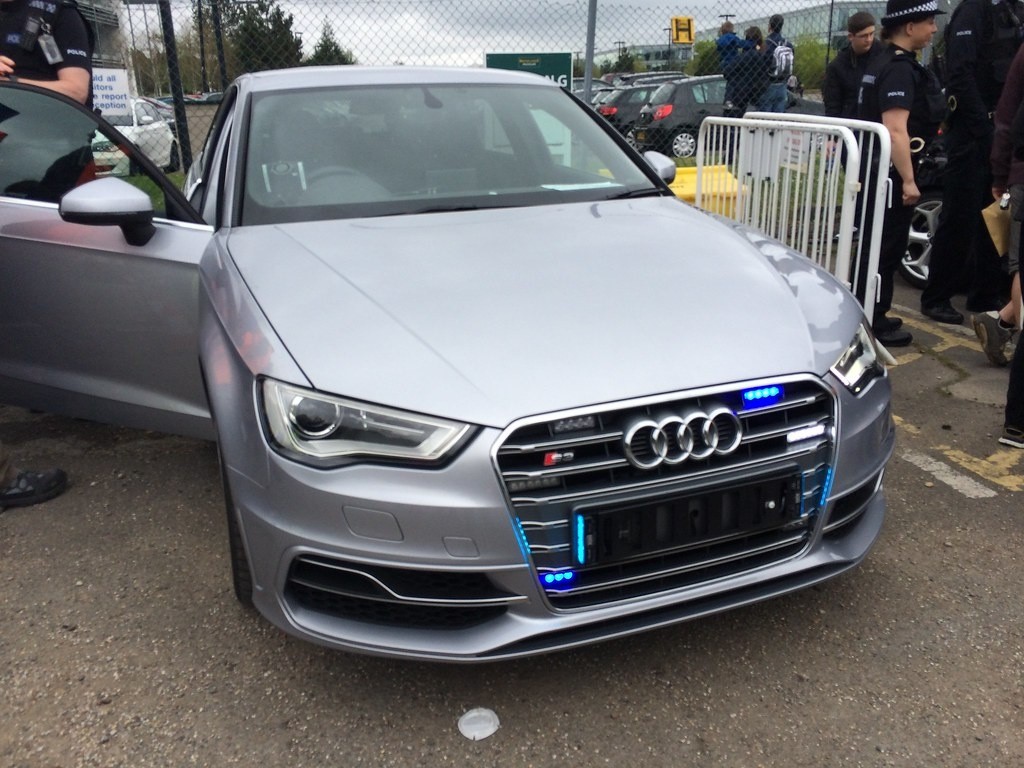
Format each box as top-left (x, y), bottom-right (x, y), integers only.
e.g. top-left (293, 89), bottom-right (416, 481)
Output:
top-left (1000, 193), bottom-right (1010, 207)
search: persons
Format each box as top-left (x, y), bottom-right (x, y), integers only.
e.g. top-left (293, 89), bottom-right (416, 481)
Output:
top-left (755, 14), bottom-right (795, 113)
top-left (0, 0), bottom-right (96, 199)
top-left (821, 0), bottom-right (1024, 448)
top-left (723, 26), bottom-right (767, 165)
top-left (0, 441), bottom-right (67, 507)
top-left (716, 21), bottom-right (761, 113)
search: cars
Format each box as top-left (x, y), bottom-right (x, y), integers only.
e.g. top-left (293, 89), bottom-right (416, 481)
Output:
top-left (90, 98), bottom-right (181, 179)
top-left (141, 96), bottom-right (177, 138)
top-left (569, 72), bottom-right (727, 159)
top-left (898, 125), bottom-right (949, 292)
top-left (156, 89), bottom-right (224, 103)
top-left (0, 64), bottom-right (896, 665)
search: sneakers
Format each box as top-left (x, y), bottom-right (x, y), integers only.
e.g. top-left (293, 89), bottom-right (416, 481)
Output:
top-left (974, 310), bottom-right (1019, 367)
top-left (998, 422), bottom-right (1024, 448)
top-left (0, 469), bottom-right (67, 513)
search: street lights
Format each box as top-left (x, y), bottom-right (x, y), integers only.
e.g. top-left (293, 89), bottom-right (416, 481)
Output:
top-left (573, 51), bottom-right (583, 67)
top-left (663, 28), bottom-right (673, 71)
top-left (613, 41), bottom-right (626, 62)
top-left (719, 14), bottom-right (737, 22)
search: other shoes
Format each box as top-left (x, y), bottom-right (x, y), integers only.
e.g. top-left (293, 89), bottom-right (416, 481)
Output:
top-left (722, 102), bottom-right (740, 110)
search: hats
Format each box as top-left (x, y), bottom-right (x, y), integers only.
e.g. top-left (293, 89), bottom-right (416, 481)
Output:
top-left (881, 0), bottom-right (948, 27)
top-left (771, 14), bottom-right (783, 33)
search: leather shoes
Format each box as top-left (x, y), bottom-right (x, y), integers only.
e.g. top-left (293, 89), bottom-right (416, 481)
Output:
top-left (886, 318), bottom-right (902, 329)
top-left (878, 331), bottom-right (912, 347)
top-left (967, 299), bottom-right (1005, 312)
top-left (921, 301), bottom-right (964, 324)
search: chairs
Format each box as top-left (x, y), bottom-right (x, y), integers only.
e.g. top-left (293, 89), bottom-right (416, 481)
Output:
top-left (273, 112), bottom-right (342, 193)
top-left (418, 109), bottom-right (486, 188)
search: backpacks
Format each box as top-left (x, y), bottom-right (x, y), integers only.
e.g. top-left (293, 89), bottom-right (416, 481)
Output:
top-left (766, 38), bottom-right (793, 78)
